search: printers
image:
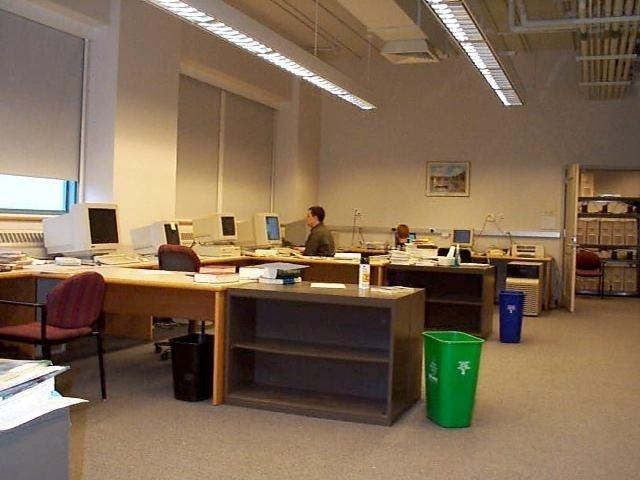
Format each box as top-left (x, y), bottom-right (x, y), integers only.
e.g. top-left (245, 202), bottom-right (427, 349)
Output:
top-left (511, 244), bottom-right (545, 258)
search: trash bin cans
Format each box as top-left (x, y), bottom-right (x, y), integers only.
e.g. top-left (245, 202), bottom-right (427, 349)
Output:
top-left (169, 334), bottom-right (214, 402)
top-left (498, 290), bottom-right (527, 343)
top-left (421, 331), bottom-right (484, 428)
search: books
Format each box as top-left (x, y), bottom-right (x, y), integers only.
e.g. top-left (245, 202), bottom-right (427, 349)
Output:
top-left (195, 262), bottom-right (303, 285)
top-left (0, 250), bottom-right (33, 265)
top-left (369, 252), bottom-right (436, 267)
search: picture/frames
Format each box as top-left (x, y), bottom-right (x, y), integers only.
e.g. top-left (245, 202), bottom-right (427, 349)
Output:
top-left (425, 159), bottom-right (472, 199)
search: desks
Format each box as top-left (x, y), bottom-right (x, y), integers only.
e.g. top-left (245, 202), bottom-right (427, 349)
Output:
top-left (95, 243), bottom-right (555, 339)
top-left (0, 258), bottom-right (225, 410)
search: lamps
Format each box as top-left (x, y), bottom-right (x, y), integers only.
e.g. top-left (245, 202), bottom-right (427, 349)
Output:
top-left (138, 0), bottom-right (383, 114)
top-left (424, 0), bottom-right (526, 109)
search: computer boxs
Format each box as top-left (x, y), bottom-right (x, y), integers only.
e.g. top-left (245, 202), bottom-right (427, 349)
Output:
top-left (192, 245), bottom-right (241, 256)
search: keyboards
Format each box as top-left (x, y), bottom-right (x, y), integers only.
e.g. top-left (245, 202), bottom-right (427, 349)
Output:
top-left (93, 254), bottom-right (141, 264)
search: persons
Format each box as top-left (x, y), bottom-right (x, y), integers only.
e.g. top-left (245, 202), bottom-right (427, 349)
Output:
top-left (394, 225), bottom-right (410, 248)
top-left (292, 206), bottom-right (336, 257)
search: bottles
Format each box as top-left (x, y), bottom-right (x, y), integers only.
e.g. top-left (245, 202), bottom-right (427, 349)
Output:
top-left (358, 257), bottom-right (371, 289)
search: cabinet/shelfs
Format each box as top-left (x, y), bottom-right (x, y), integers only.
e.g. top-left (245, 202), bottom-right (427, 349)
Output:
top-left (220, 281), bottom-right (428, 427)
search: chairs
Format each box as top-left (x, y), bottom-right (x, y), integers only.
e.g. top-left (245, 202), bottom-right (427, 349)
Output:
top-left (575, 250), bottom-right (607, 299)
top-left (1, 270), bottom-right (112, 400)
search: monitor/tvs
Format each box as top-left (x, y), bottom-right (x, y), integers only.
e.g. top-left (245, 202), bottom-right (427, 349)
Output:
top-left (452, 228), bottom-right (473, 247)
top-left (41, 202), bottom-right (120, 263)
top-left (407, 233), bottom-right (416, 242)
top-left (195, 215), bottom-right (237, 244)
top-left (131, 221), bottom-right (181, 256)
top-left (238, 213), bottom-right (282, 248)
